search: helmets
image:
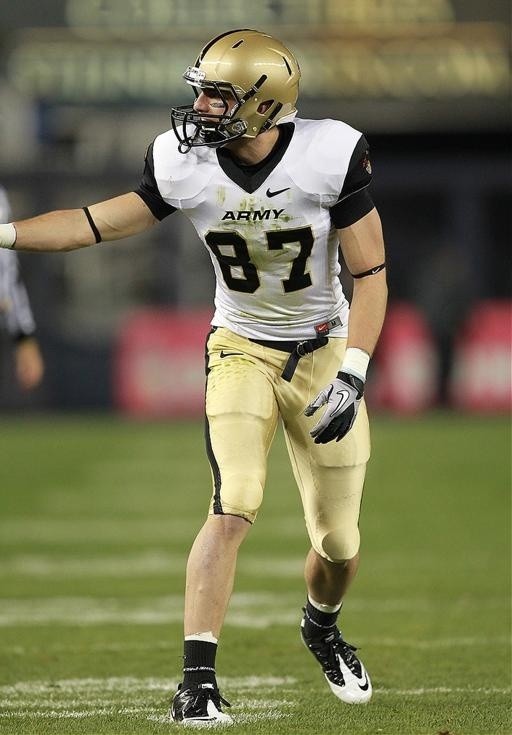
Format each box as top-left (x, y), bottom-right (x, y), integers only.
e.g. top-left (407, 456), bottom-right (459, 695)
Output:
top-left (181, 26), bottom-right (303, 142)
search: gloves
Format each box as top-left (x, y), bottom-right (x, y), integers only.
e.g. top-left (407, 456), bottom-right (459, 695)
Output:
top-left (303, 369), bottom-right (367, 446)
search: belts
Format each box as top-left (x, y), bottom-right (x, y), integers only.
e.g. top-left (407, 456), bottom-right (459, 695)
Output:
top-left (246, 335), bottom-right (331, 383)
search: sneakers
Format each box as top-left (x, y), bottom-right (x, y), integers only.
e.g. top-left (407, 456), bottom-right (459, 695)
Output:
top-left (168, 680), bottom-right (237, 727)
top-left (299, 605), bottom-right (377, 707)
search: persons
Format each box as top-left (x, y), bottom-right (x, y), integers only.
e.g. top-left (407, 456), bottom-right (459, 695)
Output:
top-left (0, 26), bottom-right (391, 727)
top-left (0, 187), bottom-right (45, 389)
